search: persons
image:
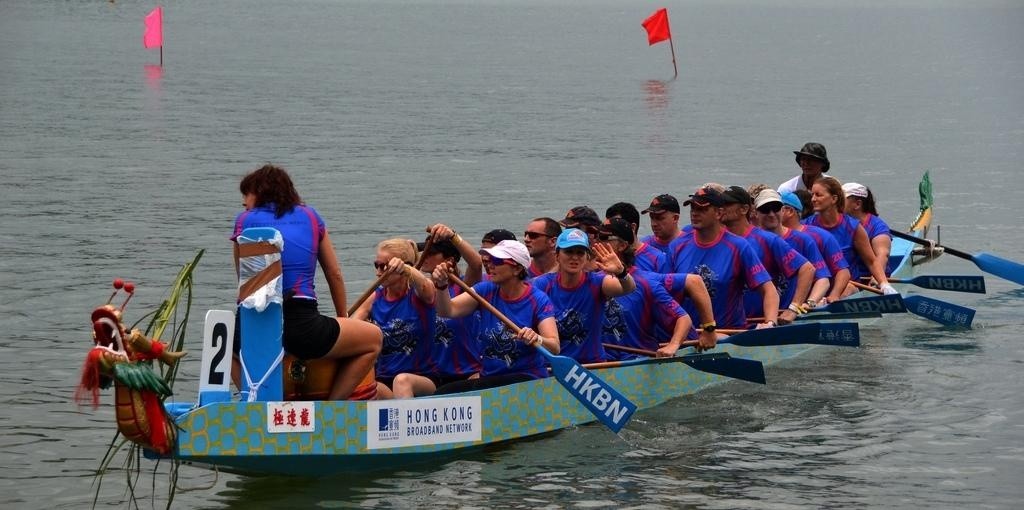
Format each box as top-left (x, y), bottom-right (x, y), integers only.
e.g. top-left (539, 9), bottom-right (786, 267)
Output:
top-left (640, 141), bottom-right (900, 342)
top-left (349, 200), bottom-right (718, 400)
top-left (231, 163), bottom-right (384, 402)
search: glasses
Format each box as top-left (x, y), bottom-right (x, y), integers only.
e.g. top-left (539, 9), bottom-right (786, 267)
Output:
top-left (598, 233), bottom-right (625, 243)
top-left (524, 231), bottom-right (552, 239)
top-left (374, 261), bottom-right (413, 269)
top-left (488, 255), bottom-right (518, 266)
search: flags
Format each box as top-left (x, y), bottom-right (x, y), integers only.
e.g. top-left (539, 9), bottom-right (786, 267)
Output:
top-left (641, 8), bottom-right (671, 45)
top-left (143, 7), bottom-right (162, 48)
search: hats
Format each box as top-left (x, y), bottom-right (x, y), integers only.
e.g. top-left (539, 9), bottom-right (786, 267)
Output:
top-left (587, 218), bottom-right (634, 245)
top-left (842, 182), bottom-right (868, 198)
top-left (416, 235), bottom-right (461, 262)
top-left (482, 229), bottom-right (517, 244)
top-left (683, 183), bottom-right (803, 212)
top-left (555, 228), bottom-right (590, 253)
top-left (793, 143), bottom-right (830, 172)
top-left (641, 194), bottom-right (680, 214)
top-left (559, 206), bottom-right (602, 228)
top-left (478, 240), bottom-right (531, 270)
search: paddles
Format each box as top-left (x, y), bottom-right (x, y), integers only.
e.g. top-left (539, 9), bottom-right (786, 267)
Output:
top-left (448, 272), bottom-right (637, 433)
top-left (601, 343), bottom-right (766, 385)
top-left (658, 323), bottom-right (861, 348)
top-left (890, 228), bottom-right (1024, 294)
top-left (849, 280), bottom-right (976, 326)
top-left (742, 310), bottom-right (884, 323)
top-left (776, 293), bottom-right (909, 316)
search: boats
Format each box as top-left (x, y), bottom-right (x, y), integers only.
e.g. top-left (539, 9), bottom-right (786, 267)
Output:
top-left (83, 169), bottom-right (934, 474)
